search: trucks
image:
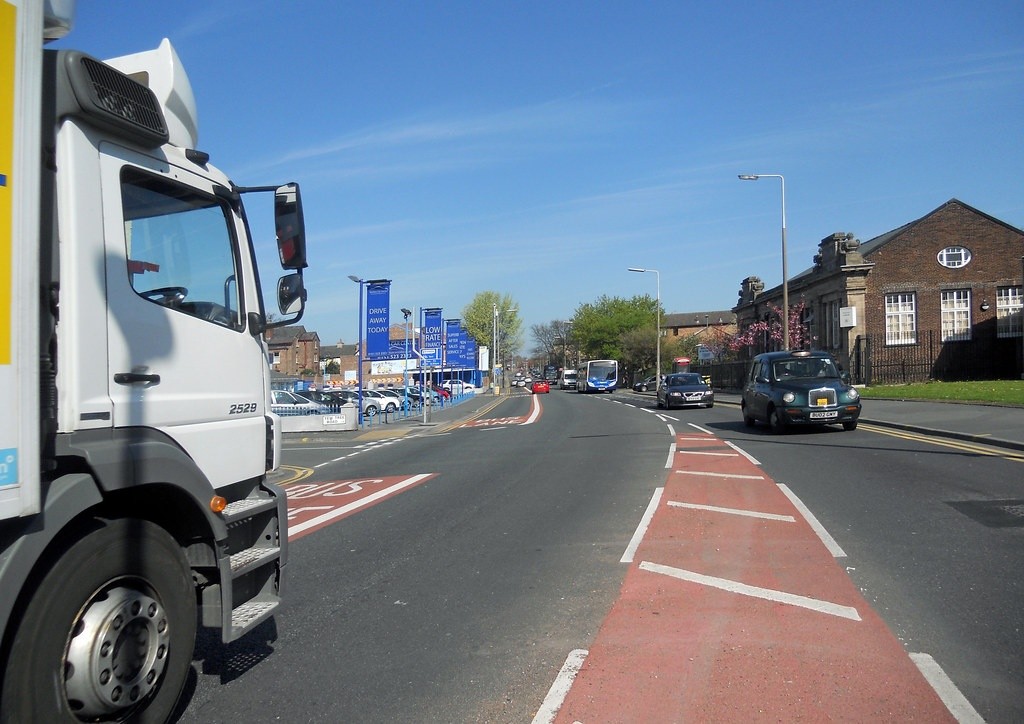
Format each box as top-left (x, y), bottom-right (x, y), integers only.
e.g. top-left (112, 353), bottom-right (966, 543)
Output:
top-left (543, 365), bottom-right (559, 385)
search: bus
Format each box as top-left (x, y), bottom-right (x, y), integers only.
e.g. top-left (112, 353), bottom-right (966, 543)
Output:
top-left (576, 360), bottom-right (618, 394)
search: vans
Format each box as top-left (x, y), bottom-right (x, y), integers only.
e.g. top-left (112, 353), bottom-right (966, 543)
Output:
top-left (559, 370), bottom-right (578, 391)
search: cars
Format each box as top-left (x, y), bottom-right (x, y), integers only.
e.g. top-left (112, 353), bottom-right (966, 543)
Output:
top-left (360, 389), bottom-right (400, 414)
top-left (633, 372), bottom-right (666, 392)
top-left (278, 390), bottom-right (347, 413)
top-left (440, 380), bottom-right (475, 392)
top-left (270, 389), bottom-right (331, 416)
top-left (406, 384), bottom-right (453, 406)
top-left (657, 373), bottom-right (714, 410)
top-left (507, 364), bottom-right (542, 387)
top-left (332, 391), bottom-right (381, 416)
top-left (740, 351), bottom-right (862, 432)
top-left (532, 380), bottom-right (549, 393)
top-left (392, 390), bottom-right (424, 408)
top-left (376, 390), bottom-right (414, 410)
top-left (703, 375), bottom-right (710, 384)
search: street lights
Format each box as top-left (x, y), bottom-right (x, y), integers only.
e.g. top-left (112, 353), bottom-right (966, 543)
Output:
top-left (738, 173), bottom-right (789, 350)
top-left (348, 275), bottom-right (364, 423)
top-left (496, 309), bottom-right (517, 385)
top-left (563, 320), bottom-right (574, 369)
top-left (706, 315), bottom-right (709, 340)
top-left (628, 268), bottom-right (661, 391)
top-left (401, 309), bottom-right (412, 415)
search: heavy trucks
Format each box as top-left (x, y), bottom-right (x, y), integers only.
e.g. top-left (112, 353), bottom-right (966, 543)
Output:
top-left (1, 0), bottom-right (308, 724)
